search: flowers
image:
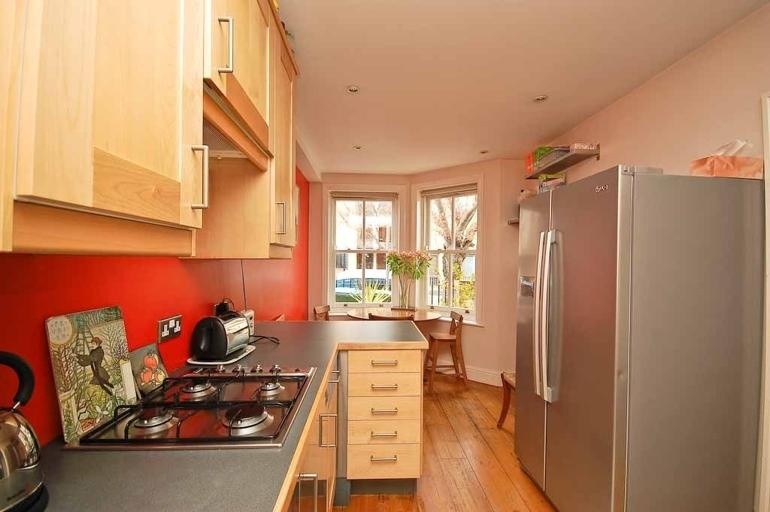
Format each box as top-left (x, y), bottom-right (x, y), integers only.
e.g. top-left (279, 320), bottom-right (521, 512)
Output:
top-left (384, 247), bottom-right (432, 295)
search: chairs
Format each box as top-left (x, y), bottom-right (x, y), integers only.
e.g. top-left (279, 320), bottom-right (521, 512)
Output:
top-left (314, 304), bottom-right (330, 321)
top-left (497, 370), bottom-right (516, 429)
top-left (424, 312), bottom-right (469, 395)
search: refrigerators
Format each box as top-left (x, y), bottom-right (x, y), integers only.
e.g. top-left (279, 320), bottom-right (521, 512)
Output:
top-left (515, 165), bottom-right (766, 512)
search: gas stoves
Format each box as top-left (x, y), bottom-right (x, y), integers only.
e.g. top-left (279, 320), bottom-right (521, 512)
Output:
top-left (62, 363), bottom-right (317, 450)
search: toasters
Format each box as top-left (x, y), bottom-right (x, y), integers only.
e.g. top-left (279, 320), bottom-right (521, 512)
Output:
top-left (191, 309), bottom-right (251, 361)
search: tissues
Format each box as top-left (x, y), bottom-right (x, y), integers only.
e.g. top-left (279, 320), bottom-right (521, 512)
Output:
top-left (689, 138), bottom-right (764, 178)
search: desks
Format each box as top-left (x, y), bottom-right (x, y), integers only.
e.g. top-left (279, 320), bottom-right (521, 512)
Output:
top-left (346, 307), bottom-right (442, 322)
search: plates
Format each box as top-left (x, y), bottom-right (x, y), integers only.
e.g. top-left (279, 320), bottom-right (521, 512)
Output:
top-left (186, 345), bottom-right (256, 366)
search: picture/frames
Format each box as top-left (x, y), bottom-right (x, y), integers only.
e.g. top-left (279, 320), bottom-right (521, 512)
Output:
top-left (46, 306), bottom-right (137, 444)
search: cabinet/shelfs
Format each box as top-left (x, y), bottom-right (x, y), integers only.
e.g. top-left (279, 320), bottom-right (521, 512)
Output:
top-left (339, 321), bottom-right (429, 497)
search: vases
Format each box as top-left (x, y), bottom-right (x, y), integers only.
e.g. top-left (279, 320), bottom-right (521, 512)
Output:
top-left (399, 285), bottom-right (409, 310)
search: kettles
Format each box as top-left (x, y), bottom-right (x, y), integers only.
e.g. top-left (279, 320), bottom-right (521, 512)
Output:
top-left (0, 350), bottom-right (51, 512)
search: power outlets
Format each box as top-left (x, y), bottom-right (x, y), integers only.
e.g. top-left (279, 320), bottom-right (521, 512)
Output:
top-left (161, 315), bottom-right (183, 340)
